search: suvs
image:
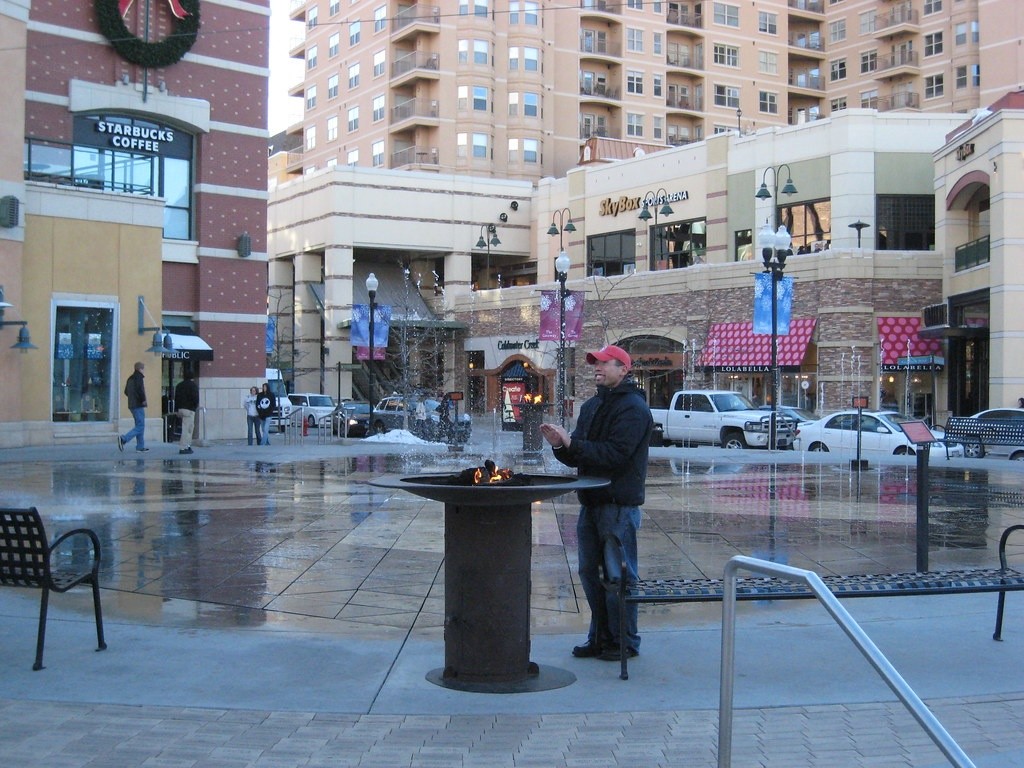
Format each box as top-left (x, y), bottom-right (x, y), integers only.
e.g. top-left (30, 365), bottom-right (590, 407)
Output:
top-left (372, 394), bottom-right (471, 444)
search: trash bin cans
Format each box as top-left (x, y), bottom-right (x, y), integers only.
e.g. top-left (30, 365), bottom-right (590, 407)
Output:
top-left (649, 422), bottom-right (664, 448)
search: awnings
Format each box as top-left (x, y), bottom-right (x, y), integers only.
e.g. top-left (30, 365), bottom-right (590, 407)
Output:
top-left (695, 319), bottom-right (817, 372)
top-left (163, 326), bottom-right (214, 362)
top-left (878, 317), bottom-right (942, 374)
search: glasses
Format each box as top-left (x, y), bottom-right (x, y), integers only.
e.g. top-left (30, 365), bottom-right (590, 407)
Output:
top-left (593, 361), bottom-right (620, 367)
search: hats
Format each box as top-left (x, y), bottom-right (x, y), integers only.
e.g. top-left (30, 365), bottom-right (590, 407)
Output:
top-left (585, 345), bottom-right (631, 372)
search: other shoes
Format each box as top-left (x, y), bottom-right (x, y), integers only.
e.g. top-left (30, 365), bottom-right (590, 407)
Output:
top-left (179, 448), bottom-right (194, 454)
top-left (117, 436), bottom-right (124, 452)
top-left (135, 447), bottom-right (151, 453)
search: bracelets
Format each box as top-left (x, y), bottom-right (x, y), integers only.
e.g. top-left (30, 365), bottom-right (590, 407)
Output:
top-left (553, 445), bottom-right (564, 450)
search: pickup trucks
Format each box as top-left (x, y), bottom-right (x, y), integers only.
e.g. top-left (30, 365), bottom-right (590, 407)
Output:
top-left (648, 388), bottom-right (798, 450)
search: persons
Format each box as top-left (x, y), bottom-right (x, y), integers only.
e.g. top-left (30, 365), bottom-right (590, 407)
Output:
top-left (415, 396), bottom-right (430, 440)
top-left (541, 346), bottom-right (654, 661)
top-left (256, 383), bottom-right (276, 445)
top-left (244, 386), bottom-right (261, 445)
top-left (174, 368), bottom-right (199, 455)
top-left (118, 362), bottom-right (151, 453)
top-left (436, 395), bottom-right (453, 443)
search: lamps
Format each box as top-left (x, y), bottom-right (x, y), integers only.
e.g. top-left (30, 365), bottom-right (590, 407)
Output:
top-left (0, 287), bottom-right (41, 352)
top-left (488, 224), bottom-right (496, 233)
top-left (500, 212), bottom-right (507, 222)
top-left (511, 201), bottom-right (518, 210)
top-left (848, 220), bottom-right (870, 248)
top-left (138, 296), bottom-right (179, 357)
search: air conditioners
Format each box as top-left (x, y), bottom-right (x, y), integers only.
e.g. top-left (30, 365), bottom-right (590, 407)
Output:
top-left (433, 7), bottom-right (439, 14)
top-left (432, 147), bottom-right (439, 154)
top-left (432, 53), bottom-right (439, 60)
top-left (432, 100), bottom-right (439, 107)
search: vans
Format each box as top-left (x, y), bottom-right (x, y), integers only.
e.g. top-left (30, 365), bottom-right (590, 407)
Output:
top-left (266, 367), bottom-right (293, 433)
top-left (286, 392), bottom-right (336, 428)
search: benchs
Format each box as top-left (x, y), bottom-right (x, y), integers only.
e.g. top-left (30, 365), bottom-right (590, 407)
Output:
top-left (0, 505), bottom-right (107, 671)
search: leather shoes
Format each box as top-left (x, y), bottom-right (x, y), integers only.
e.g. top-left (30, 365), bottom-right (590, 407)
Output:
top-left (573, 640), bottom-right (603, 658)
top-left (601, 642), bottom-right (637, 661)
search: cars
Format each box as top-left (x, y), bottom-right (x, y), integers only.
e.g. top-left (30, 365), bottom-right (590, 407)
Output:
top-left (332, 399), bottom-right (376, 438)
top-left (758, 404), bottom-right (821, 449)
top-left (944, 407), bottom-right (1024, 464)
top-left (797, 408), bottom-right (965, 457)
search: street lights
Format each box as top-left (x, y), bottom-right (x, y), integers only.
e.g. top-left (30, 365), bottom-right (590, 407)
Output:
top-left (756, 216), bottom-right (792, 447)
top-left (547, 206), bottom-right (577, 250)
top-left (755, 163), bottom-right (798, 233)
top-left (554, 251), bottom-right (571, 427)
top-left (365, 273), bottom-right (380, 437)
top-left (476, 223), bottom-right (502, 289)
top-left (638, 188), bottom-right (675, 271)
